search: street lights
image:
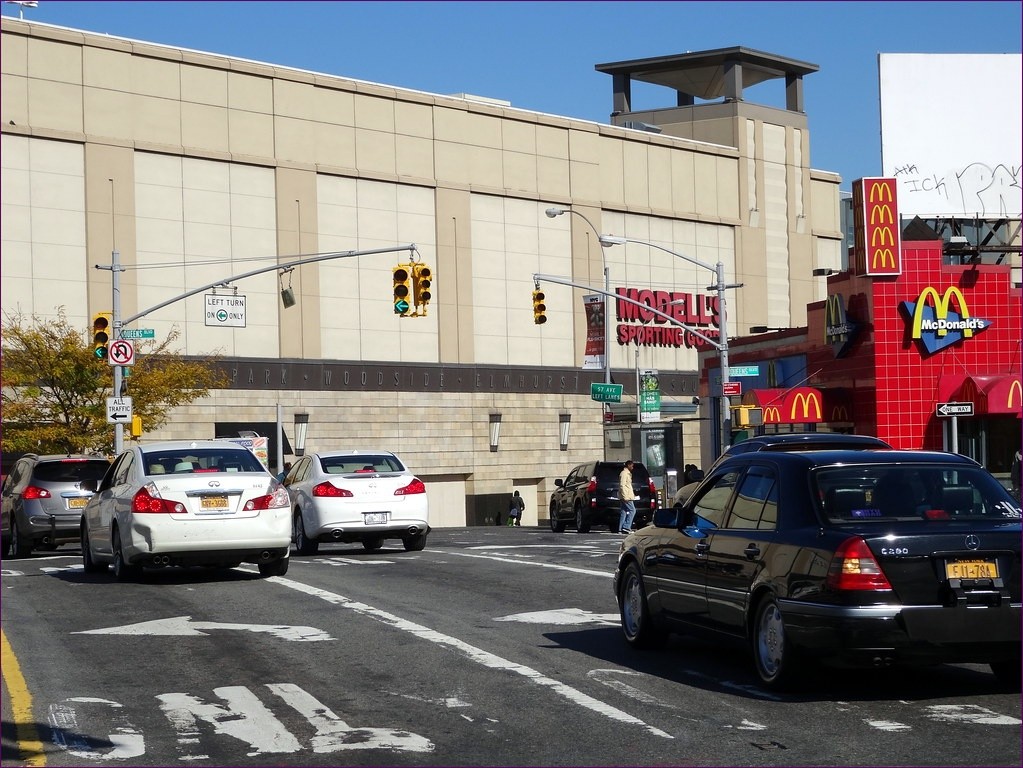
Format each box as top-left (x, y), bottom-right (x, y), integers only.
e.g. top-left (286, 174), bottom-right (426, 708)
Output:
top-left (599, 235), bottom-right (734, 457)
top-left (636, 298), bottom-right (684, 421)
top-left (545, 208), bottom-right (611, 422)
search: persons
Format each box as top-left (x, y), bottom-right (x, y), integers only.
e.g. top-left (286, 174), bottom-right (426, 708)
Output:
top-left (1011, 450), bottom-right (1023, 501)
top-left (509, 490), bottom-right (525, 526)
top-left (618, 461), bottom-right (638, 534)
top-left (682, 464), bottom-right (704, 485)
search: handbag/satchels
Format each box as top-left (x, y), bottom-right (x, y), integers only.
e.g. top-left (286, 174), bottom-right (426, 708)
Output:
top-left (509, 509), bottom-right (517, 519)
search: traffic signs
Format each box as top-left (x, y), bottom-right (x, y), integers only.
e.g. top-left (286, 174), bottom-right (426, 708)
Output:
top-left (936, 402), bottom-right (974, 417)
top-left (105, 397), bottom-right (133, 423)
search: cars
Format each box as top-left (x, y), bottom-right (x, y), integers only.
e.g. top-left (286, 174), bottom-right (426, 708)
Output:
top-left (281, 449), bottom-right (431, 557)
top-left (611, 450), bottom-right (1023, 695)
top-left (79, 440), bottom-right (293, 579)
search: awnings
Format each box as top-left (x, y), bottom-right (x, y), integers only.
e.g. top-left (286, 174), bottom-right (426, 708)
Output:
top-left (215, 422), bottom-right (293, 455)
top-left (743, 387), bottom-right (854, 424)
top-left (958, 376), bottom-right (1023, 418)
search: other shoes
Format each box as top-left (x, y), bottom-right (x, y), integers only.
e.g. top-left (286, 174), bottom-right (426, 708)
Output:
top-left (618, 531), bottom-right (628, 534)
top-left (622, 528), bottom-right (635, 533)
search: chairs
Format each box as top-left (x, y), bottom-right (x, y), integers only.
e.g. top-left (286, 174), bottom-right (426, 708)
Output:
top-left (940, 485), bottom-right (974, 516)
top-left (148, 464), bottom-right (165, 475)
top-left (826, 488), bottom-right (867, 518)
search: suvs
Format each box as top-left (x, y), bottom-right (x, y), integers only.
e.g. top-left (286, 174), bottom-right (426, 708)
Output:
top-left (550, 461), bottom-right (657, 534)
top-left (0, 452), bottom-right (111, 558)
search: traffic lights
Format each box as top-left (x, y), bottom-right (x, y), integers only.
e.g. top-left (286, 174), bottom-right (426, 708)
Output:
top-left (748, 408), bottom-right (763, 426)
top-left (393, 267), bottom-right (412, 314)
top-left (734, 408), bottom-right (749, 425)
top-left (411, 265), bottom-right (432, 307)
top-left (533, 290), bottom-right (547, 325)
top-left (92, 313), bottom-right (110, 361)
top-left (130, 415), bottom-right (142, 436)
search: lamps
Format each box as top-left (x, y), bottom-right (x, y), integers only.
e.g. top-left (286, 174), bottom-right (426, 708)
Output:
top-left (813, 269), bottom-right (843, 276)
top-left (750, 327), bottom-right (786, 333)
top-left (945, 236), bottom-right (969, 251)
